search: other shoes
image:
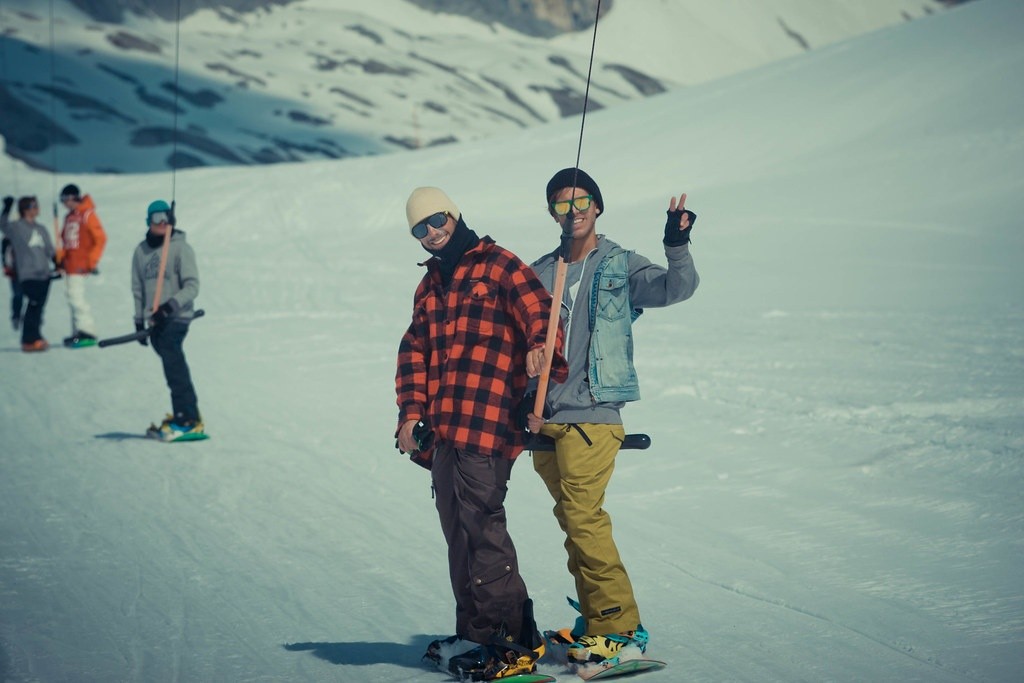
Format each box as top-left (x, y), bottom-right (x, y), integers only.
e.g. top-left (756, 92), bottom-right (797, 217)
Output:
top-left (22, 338), bottom-right (48, 351)
top-left (158, 418), bottom-right (204, 441)
top-left (11, 317), bottom-right (19, 330)
top-left (64, 332), bottom-right (97, 348)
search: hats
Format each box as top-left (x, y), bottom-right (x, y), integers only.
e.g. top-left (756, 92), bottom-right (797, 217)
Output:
top-left (60, 184), bottom-right (79, 201)
top-left (146, 200), bottom-right (176, 226)
top-left (545, 167), bottom-right (604, 220)
top-left (407, 186), bottom-right (461, 231)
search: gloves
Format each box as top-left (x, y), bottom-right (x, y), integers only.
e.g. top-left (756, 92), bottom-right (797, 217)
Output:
top-left (395, 417), bottom-right (436, 454)
top-left (135, 321), bottom-right (148, 346)
top-left (5, 266), bottom-right (12, 275)
top-left (2, 196), bottom-right (14, 213)
top-left (150, 301), bottom-right (173, 327)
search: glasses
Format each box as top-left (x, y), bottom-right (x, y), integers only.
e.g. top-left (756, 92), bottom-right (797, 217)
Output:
top-left (150, 210), bottom-right (172, 224)
top-left (411, 211), bottom-right (449, 239)
top-left (552, 194), bottom-right (594, 216)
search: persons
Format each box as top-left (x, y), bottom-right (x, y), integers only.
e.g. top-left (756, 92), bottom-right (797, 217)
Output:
top-left (60, 184), bottom-right (107, 345)
top-left (0, 194), bottom-right (59, 351)
top-left (131, 200), bottom-right (204, 441)
top-left (2, 236), bottom-right (22, 329)
top-left (395, 186), bottom-right (569, 683)
top-left (516, 167), bottom-right (700, 669)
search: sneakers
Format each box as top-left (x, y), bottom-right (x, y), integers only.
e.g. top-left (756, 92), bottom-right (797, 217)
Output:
top-left (448, 630), bottom-right (548, 679)
top-left (567, 614), bottom-right (650, 674)
top-left (428, 632), bottom-right (482, 672)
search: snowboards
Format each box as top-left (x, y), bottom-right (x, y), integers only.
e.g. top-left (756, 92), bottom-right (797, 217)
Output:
top-left (426, 636), bottom-right (557, 682)
top-left (544, 628), bottom-right (667, 682)
top-left (146, 417), bottom-right (210, 442)
top-left (69, 338), bottom-right (97, 347)
